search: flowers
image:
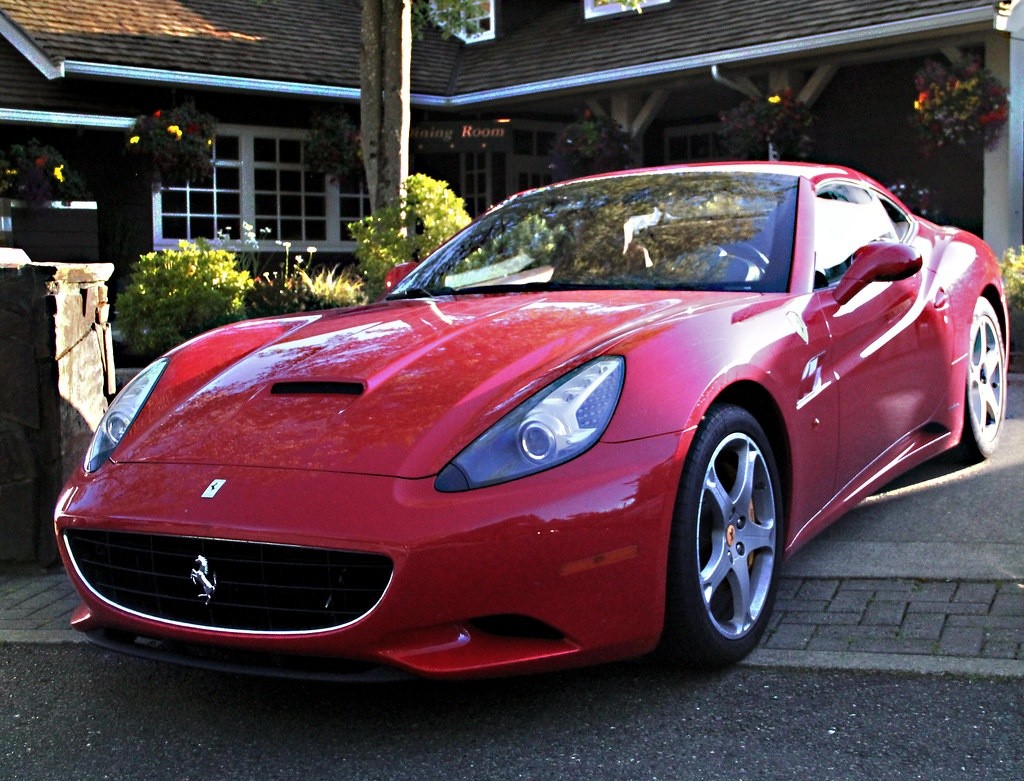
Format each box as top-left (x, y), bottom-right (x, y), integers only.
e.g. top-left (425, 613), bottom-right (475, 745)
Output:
top-left (715, 92), bottom-right (816, 158)
top-left (912, 58), bottom-right (1008, 158)
top-left (5, 137), bottom-right (88, 204)
top-left (127, 106), bottom-right (214, 192)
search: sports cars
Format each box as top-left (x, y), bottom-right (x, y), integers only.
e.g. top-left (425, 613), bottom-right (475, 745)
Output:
top-left (52, 161), bottom-right (1010, 684)
